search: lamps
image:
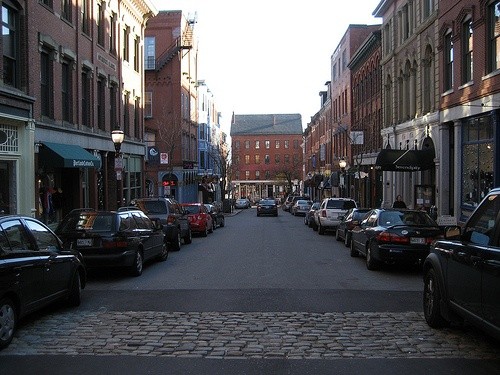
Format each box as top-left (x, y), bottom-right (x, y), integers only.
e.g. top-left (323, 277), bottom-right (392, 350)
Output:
top-left (96, 124), bottom-right (123, 160)
top-left (340, 159), bottom-right (358, 176)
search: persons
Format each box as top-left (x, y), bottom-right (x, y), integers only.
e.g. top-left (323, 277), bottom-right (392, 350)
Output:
top-left (393, 196), bottom-right (407, 208)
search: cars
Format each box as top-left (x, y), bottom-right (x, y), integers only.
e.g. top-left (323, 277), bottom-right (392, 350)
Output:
top-left (350, 208), bottom-right (445, 271)
top-left (304, 202), bottom-right (322, 228)
top-left (292, 199), bottom-right (314, 216)
top-left (0, 212), bottom-right (88, 348)
top-left (54, 206), bottom-right (168, 277)
top-left (173, 202), bottom-right (214, 237)
top-left (255, 199), bottom-right (279, 217)
top-left (204, 204), bottom-right (225, 231)
top-left (335, 209), bottom-right (374, 247)
top-left (234, 198), bottom-right (251, 209)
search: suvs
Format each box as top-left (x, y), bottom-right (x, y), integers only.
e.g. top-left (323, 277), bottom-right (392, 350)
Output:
top-left (128, 197), bottom-right (192, 250)
top-left (282, 196), bottom-right (304, 214)
top-left (421, 188), bottom-right (500, 336)
top-left (311, 197), bottom-right (358, 234)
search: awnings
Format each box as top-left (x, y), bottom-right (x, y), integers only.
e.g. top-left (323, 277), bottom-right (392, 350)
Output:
top-left (37, 140), bottom-right (101, 169)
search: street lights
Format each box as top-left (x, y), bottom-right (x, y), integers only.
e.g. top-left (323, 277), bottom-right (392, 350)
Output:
top-left (338, 157), bottom-right (347, 198)
top-left (110, 122), bottom-right (125, 208)
top-left (307, 173), bottom-right (313, 201)
